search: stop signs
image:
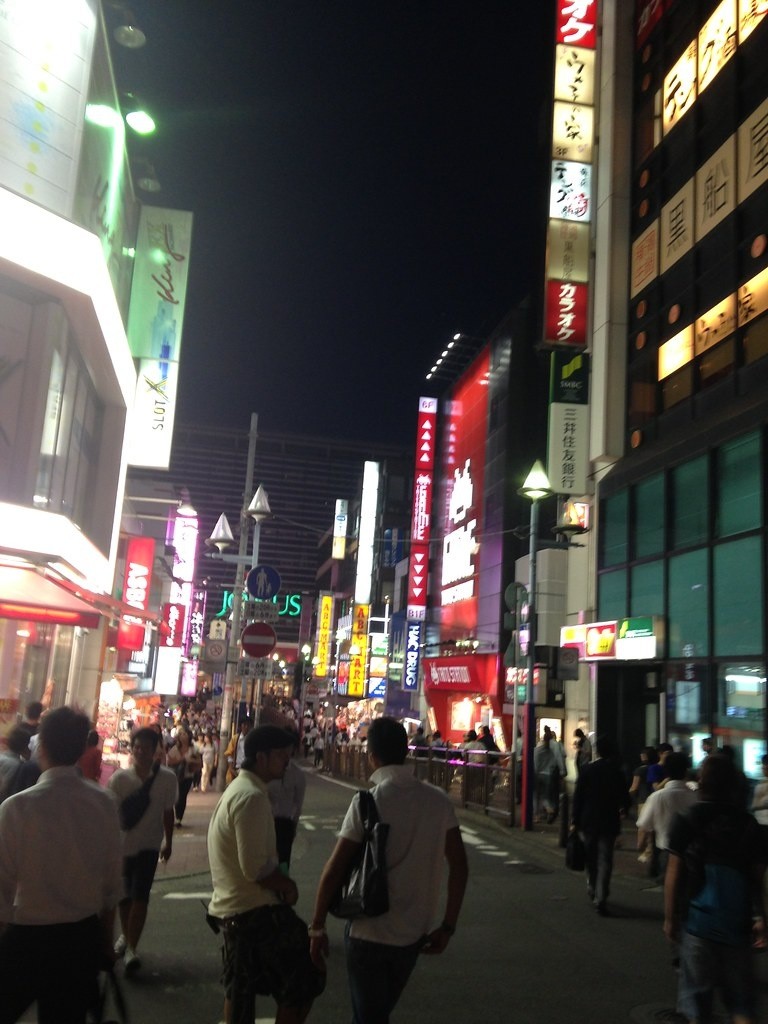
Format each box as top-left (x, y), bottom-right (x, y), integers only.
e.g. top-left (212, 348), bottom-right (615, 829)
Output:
top-left (240, 622), bottom-right (277, 659)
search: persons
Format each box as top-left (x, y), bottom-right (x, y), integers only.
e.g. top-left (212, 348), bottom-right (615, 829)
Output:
top-left (127, 698), bottom-right (252, 794)
top-left (278, 699), bottom-right (371, 767)
top-left (267, 729), bottom-right (306, 877)
top-left (207, 726), bottom-right (312, 1024)
top-left (0, 702), bottom-right (179, 1024)
top-left (568, 736), bottom-right (768, 1024)
top-left (308, 716), bottom-right (469, 1024)
top-left (407, 724), bottom-right (593, 823)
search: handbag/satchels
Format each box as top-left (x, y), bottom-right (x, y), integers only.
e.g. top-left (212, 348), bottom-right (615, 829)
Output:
top-left (119, 761), bottom-right (160, 830)
top-left (565, 832), bottom-right (585, 870)
top-left (328, 790), bottom-right (389, 919)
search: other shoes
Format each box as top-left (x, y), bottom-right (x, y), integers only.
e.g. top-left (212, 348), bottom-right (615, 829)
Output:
top-left (638, 853), bottom-right (648, 863)
top-left (113, 934), bottom-right (126, 956)
top-left (595, 900), bottom-right (606, 911)
top-left (124, 950), bottom-right (140, 976)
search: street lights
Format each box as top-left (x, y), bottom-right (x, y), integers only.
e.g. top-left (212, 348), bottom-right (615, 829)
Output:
top-left (518, 456), bottom-right (554, 830)
top-left (203, 483), bottom-right (271, 730)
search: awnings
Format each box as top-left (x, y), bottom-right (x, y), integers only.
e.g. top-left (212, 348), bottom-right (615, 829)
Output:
top-left (0, 564), bottom-right (103, 629)
